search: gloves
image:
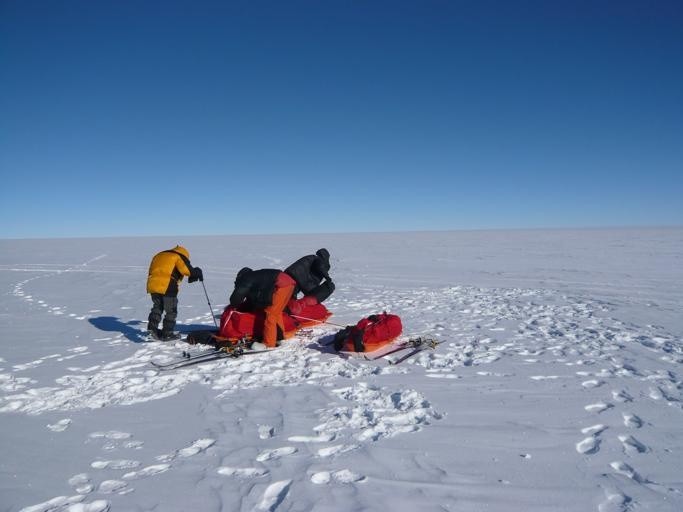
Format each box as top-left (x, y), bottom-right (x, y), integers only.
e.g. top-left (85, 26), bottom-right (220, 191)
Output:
top-left (188, 267), bottom-right (202, 284)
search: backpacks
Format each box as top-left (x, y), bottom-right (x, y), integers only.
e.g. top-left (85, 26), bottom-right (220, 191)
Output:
top-left (333, 313), bottom-right (403, 352)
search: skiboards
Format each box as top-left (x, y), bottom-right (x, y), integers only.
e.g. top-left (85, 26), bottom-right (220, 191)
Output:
top-left (150, 340), bottom-right (272, 370)
top-left (364, 337), bottom-right (447, 365)
top-left (135, 330), bottom-right (188, 343)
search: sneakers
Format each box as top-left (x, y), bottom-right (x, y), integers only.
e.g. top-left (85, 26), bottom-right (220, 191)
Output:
top-left (162, 332), bottom-right (181, 341)
top-left (147, 329), bottom-right (162, 340)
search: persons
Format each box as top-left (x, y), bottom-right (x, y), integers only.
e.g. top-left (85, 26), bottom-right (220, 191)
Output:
top-left (283, 248), bottom-right (331, 298)
top-left (145, 245), bottom-right (202, 340)
top-left (229, 267), bottom-right (296, 350)
